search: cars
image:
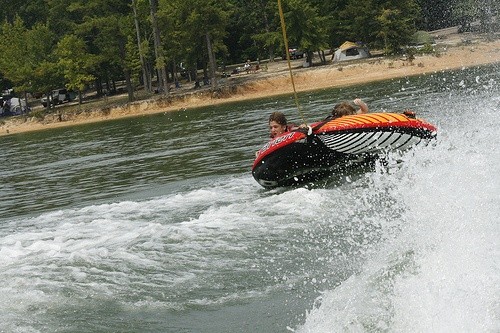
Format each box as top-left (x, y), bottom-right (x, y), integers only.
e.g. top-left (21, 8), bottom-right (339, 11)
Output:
top-left (282, 48), bottom-right (303, 60)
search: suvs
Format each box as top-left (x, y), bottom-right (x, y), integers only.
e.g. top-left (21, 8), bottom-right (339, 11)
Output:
top-left (41, 89), bottom-right (77, 107)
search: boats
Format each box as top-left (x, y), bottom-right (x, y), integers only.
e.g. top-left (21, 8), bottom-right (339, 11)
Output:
top-left (251, 112), bottom-right (439, 189)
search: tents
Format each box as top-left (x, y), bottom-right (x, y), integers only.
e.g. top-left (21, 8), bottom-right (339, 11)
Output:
top-left (406, 31), bottom-right (437, 50)
top-left (0, 97), bottom-right (32, 117)
top-left (331, 40), bottom-right (371, 62)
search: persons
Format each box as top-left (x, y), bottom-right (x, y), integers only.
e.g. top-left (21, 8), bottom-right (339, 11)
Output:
top-left (153, 72), bottom-right (229, 94)
top-left (236, 55), bottom-right (276, 75)
top-left (332, 100), bottom-right (369, 117)
top-left (268, 112), bottom-right (307, 140)
